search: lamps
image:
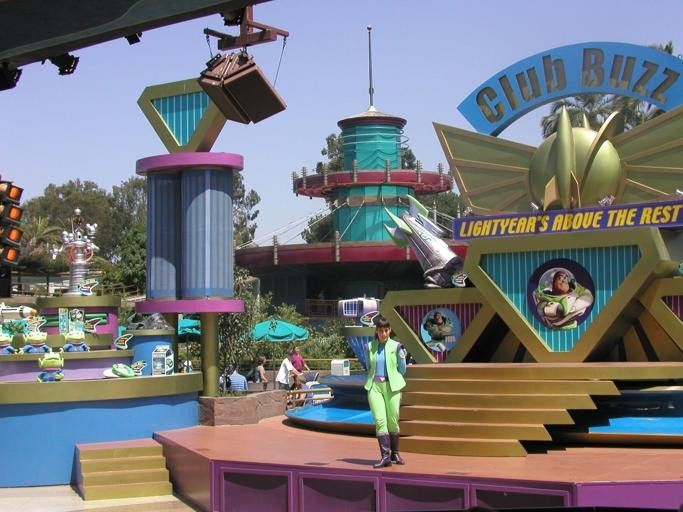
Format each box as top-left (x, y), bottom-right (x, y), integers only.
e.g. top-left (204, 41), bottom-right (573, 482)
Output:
top-left (0, 176), bottom-right (25, 268)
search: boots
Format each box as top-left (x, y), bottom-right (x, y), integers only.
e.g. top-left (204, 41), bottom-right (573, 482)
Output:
top-left (372, 431), bottom-right (391, 468)
top-left (390, 432), bottom-right (405, 466)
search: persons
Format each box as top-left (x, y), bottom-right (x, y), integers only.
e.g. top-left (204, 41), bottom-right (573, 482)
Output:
top-left (226, 361), bottom-right (248, 390)
top-left (292, 346), bottom-right (311, 380)
top-left (275, 350), bottom-right (302, 390)
top-left (365, 319), bottom-right (407, 469)
top-left (286, 375), bottom-right (312, 410)
top-left (184, 360), bottom-right (193, 373)
top-left (254, 355), bottom-right (268, 384)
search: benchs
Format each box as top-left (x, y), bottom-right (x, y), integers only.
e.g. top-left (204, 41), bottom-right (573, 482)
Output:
top-left (224, 388), bottom-right (272, 395)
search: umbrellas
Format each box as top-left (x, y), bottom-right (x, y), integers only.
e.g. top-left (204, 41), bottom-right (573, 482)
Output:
top-left (240, 321), bottom-right (310, 388)
top-left (177, 317), bottom-right (224, 372)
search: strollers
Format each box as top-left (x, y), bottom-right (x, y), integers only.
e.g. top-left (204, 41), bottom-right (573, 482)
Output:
top-left (293, 370), bottom-right (320, 389)
